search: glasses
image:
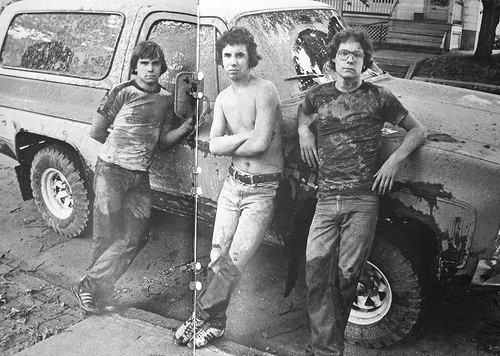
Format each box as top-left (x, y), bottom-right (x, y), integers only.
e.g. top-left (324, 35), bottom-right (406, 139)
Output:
top-left (338, 49), bottom-right (366, 62)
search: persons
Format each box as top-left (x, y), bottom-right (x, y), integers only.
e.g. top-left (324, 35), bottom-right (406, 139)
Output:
top-left (69, 40), bottom-right (195, 315)
top-left (297, 29), bottom-right (426, 356)
top-left (173, 28), bottom-right (285, 349)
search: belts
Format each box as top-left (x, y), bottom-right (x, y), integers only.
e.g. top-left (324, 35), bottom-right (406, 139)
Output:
top-left (229, 165), bottom-right (284, 185)
top-left (319, 188), bottom-right (379, 195)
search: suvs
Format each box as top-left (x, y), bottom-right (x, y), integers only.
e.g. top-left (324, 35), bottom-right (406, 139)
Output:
top-left (0, 1), bottom-right (500, 352)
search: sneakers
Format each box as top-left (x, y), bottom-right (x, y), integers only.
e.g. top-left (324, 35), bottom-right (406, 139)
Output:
top-left (187, 323), bottom-right (225, 349)
top-left (71, 275), bottom-right (99, 313)
top-left (173, 315), bottom-right (206, 345)
